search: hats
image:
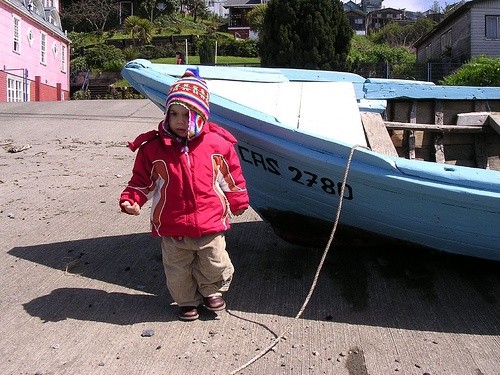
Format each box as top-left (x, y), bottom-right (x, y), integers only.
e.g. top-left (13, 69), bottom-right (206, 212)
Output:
top-left (163, 68), bottom-right (211, 141)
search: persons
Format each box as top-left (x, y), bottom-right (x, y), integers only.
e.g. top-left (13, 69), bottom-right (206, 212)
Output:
top-left (119, 68), bottom-right (249, 320)
top-left (175, 51), bottom-right (186, 65)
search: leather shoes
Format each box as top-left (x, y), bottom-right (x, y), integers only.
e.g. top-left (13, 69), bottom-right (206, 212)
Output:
top-left (205, 296), bottom-right (227, 311)
top-left (177, 305), bottom-right (199, 320)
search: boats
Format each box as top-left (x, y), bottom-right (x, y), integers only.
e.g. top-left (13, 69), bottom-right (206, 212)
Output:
top-left (120, 58), bottom-right (499, 264)
